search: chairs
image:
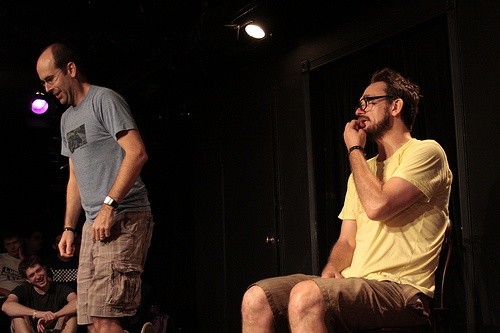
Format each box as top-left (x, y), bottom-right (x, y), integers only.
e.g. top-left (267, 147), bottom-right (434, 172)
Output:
top-left (372, 218), bottom-right (454, 333)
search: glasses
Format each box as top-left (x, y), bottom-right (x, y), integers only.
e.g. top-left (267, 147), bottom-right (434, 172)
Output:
top-left (358, 94), bottom-right (402, 111)
top-left (40, 62), bottom-right (68, 86)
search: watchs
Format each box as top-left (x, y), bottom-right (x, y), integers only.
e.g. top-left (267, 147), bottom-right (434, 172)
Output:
top-left (104, 196), bottom-right (119, 208)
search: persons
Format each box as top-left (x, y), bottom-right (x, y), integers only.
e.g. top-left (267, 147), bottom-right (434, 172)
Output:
top-left (36, 44), bottom-right (153, 333)
top-left (1, 255), bottom-right (78, 333)
top-left (241, 69), bottom-right (453, 333)
top-left (0, 234), bottom-right (29, 299)
top-left (48, 234), bottom-right (170, 333)
top-left (26, 232), bottom-right (43, 255)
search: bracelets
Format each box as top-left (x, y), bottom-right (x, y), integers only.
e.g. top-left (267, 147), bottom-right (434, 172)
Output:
top-left (33, 311), bottom-right (36, 318)
top-left (348, 146), bottom-right (366, 157)
top-left (63, 227), bottom-right (76, 236)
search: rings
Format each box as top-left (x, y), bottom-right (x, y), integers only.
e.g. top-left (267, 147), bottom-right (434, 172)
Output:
top-left (100, 235), bottom-right (104, 238)
